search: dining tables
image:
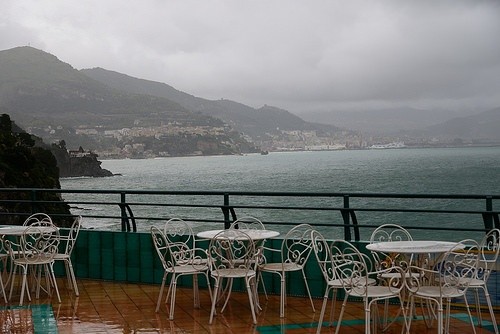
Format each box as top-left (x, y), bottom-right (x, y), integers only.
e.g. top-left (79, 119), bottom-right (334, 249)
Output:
top-left (363, 235), bottom-right (464, 334)
top-left (0, 224), bottom-right (62, 304)
top-left (195, 228), bottom-right (281, 313)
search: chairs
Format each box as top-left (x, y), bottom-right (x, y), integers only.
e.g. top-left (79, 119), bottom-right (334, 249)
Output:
top-left (16, 212), bottom-right (56, 296)
top-left (220, 214), bottom-right (268, 308)
top-left (402, 238), bottom-right (486, 332)
top-left (205, 226), bottom-right (264, 324)
top-left (161, 216), bottom-right (221, 306)
top-left (254, 224), bottom-right (319, 315)
top-left (366, 222), bottom-right (428, 324)
top-left (310, 228), bottom-right (378, 333)
top-left (148, 225), bottom-right (218, 324)
top-left (326, 237), bottom-right (411, 331)
top-left (438, 228), bottom-right (500, 334)
top-left (43, 213), bottom-right (84, 299)
top-left (8, 224), bottom-right (63, 305)
